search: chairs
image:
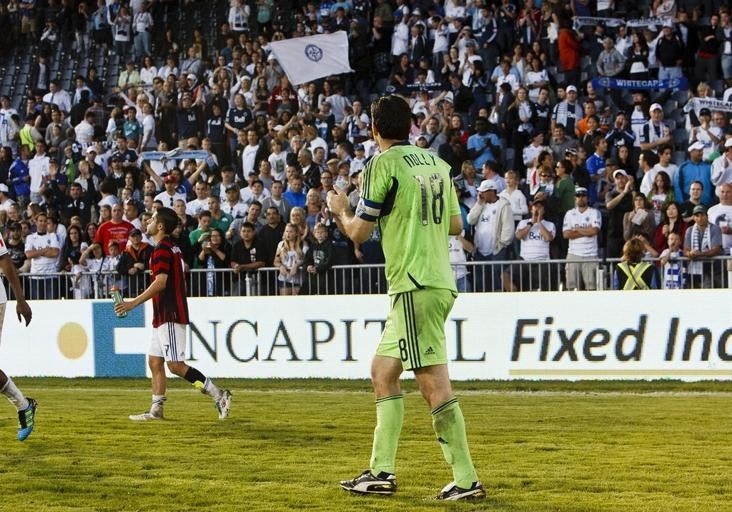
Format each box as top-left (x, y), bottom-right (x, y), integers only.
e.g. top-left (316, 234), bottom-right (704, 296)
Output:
top-left (0, 0), bottom-right (294, 113)
top-left (357, 0), bottom-right (732, 175)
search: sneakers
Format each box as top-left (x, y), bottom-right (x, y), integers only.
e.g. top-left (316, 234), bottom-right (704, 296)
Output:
top-left (434, 481), bottom-right (485, 501)
top-left (340, 470), bottom-right (396, 495)
top-left (215, 389), bottom-right (232, 419)
top-left (129, 413), bottom-right (163, 421)
top-left (17, 398), bottom-right (38, 441)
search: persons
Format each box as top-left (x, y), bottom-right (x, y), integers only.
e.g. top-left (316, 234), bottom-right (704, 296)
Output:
top-left (115, 207), bottom-right (232, 421)
top-left (1, 235), bottom-right (38, 442)
top-left (0, 1), bottom-right (731, 300)
top-left (325, 97), bottom-right (487, 499)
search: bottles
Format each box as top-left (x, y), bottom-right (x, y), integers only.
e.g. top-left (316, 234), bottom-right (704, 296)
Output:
top-left (336, 176), bottom-right (348, 196)
top-left (110, 285), bottom-right (127, 318)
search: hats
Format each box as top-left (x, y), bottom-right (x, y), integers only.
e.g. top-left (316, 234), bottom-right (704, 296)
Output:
top-left (664, 21), bottom-right (676, 28)
top-left (241, 76), bottom-right (250, 80)
top-left (187, 74), bottom-right (196, 80)
top-left (693, 205), bottom-right (706, 213)
top-left (725, 138), bottom-right (732, 148)
top-left (354, 143), bottom-right (365, 149)
top-left (6, 222), bottom-right (21, 229)
top-left (86, 145), bottom-right (97, 153)
top-left (225, 182), bottom-right (239, 190)
top-left (565, 148), bottom-right (577, 154)
top-left (440, 97), bottom-right (453, 102)
top-left (605, 157), bottom-right (619, 166)
top-left (700, 109), bottom-right (710, 114)
top-left (129, 229), bottom-right (141, 235)
top-left (477, 180), bottom-right (497, 192)
top-left (165, 175), bottom-right (176, 182)
top-left (688, 140), bottom-right (704, 152)
top-left (573, 187), bottom-right (587, 195)
top-left (596, 19), bottom-right (607, 28)
top-left (613, 169), bottom-right (627, 177)
top-left (112, 153), bottom-right (125, 161)
top-left (532, 199), bottom-right (544, 205)
top-left (649, 103), bottom-right (662, 111)
top-left (566, 85), bottom-right (577, 93)
top-left (465, 39), bottom-right (473, 46)
top-left (1, 183), bottom-right (9, 191)
top-left (617, 109), bottom-right (627, 120)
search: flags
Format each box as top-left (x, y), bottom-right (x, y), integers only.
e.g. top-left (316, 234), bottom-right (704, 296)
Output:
top-left (271, 30), bottom-right (355, 85)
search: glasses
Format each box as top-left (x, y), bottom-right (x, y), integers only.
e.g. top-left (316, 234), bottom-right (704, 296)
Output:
top-left (541, 175), bottom-right (551, 178)
